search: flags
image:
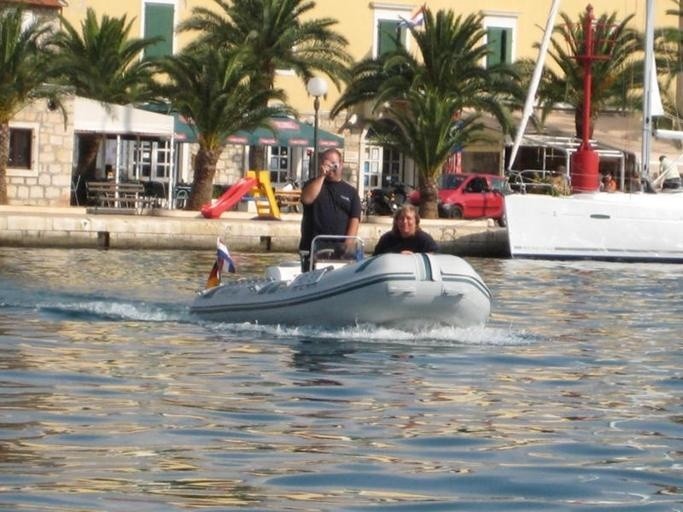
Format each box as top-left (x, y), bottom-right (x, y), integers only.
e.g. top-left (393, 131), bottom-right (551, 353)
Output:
top-left (204, 239), bottom-right (236, 290)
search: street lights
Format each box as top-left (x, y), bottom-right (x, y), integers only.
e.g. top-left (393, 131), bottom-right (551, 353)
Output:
top-left (307, 77), bottom-right (327, 178)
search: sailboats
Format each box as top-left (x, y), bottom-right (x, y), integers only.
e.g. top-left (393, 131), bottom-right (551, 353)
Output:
top-left (499, 0), bottom-right (683, 264)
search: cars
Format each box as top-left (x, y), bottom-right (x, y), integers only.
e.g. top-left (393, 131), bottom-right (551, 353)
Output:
top-left (407, 173), bottom-right (516, 228)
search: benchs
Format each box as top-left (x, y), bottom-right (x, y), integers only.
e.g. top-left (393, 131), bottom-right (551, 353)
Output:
top-left (272, 188), bottom-right (306, 211)
top-left (82, 179), bottom-right (156, 216)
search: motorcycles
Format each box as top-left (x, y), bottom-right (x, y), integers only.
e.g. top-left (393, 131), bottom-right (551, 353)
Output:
top-left (371, 175), bottom-right (407, 216)
top-left (272, 175), bottom-right (302, 213)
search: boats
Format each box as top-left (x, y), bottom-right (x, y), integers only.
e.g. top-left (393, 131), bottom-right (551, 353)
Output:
top-left (189, 234), bottom-right (493, 331)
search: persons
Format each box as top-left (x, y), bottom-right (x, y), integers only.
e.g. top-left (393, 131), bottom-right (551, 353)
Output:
top-left (552, 165), bottom-right (571, 197)
top-left (375, 203), bottom-right (437, 255)
top-left (659, 155), bottom-right (681, 189)
top-left (601, 169), bottom-right (617, 192)
top-left (299, 149), bottom-right (362, 273)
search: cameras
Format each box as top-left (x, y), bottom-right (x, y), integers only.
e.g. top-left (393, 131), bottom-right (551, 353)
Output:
top-left (329, 164), bottom-right (339, 170)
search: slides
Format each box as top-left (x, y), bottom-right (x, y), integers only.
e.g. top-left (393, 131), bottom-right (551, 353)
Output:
top-left (201, 177), bottom-right (260, 217)
